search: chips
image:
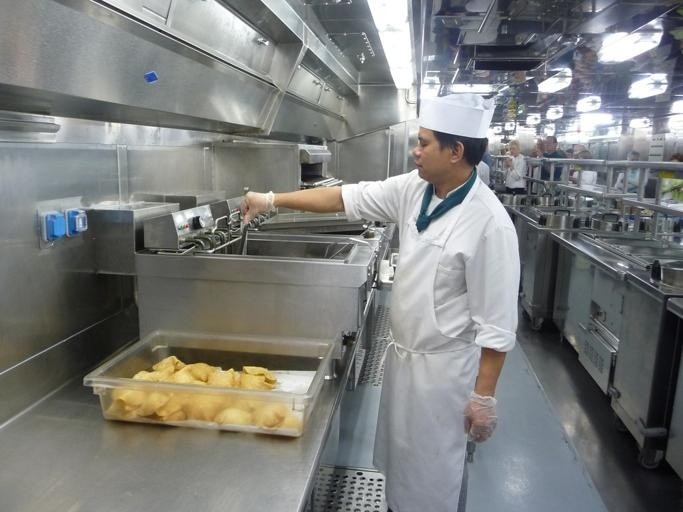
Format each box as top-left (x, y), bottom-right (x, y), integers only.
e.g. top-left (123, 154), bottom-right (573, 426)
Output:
top-left (104, 355), bottom-right (301, 431)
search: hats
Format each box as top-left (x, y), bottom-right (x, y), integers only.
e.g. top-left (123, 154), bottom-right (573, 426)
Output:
top-left (418, 89), bottom-right (497, 141)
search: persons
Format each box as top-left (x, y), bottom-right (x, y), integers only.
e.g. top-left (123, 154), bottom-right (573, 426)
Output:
top-left (615, 152), bottom-right (648, 197)
top-left (239, 91), bottom-right (520, 511)
top-left (478, 133), bottom-right (598, 196)
top-left (658, 153), bottom-right (683, 203)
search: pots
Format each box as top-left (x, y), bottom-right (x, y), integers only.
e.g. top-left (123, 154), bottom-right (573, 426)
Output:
top-left (501, 190), bottom-right (625, 233)
